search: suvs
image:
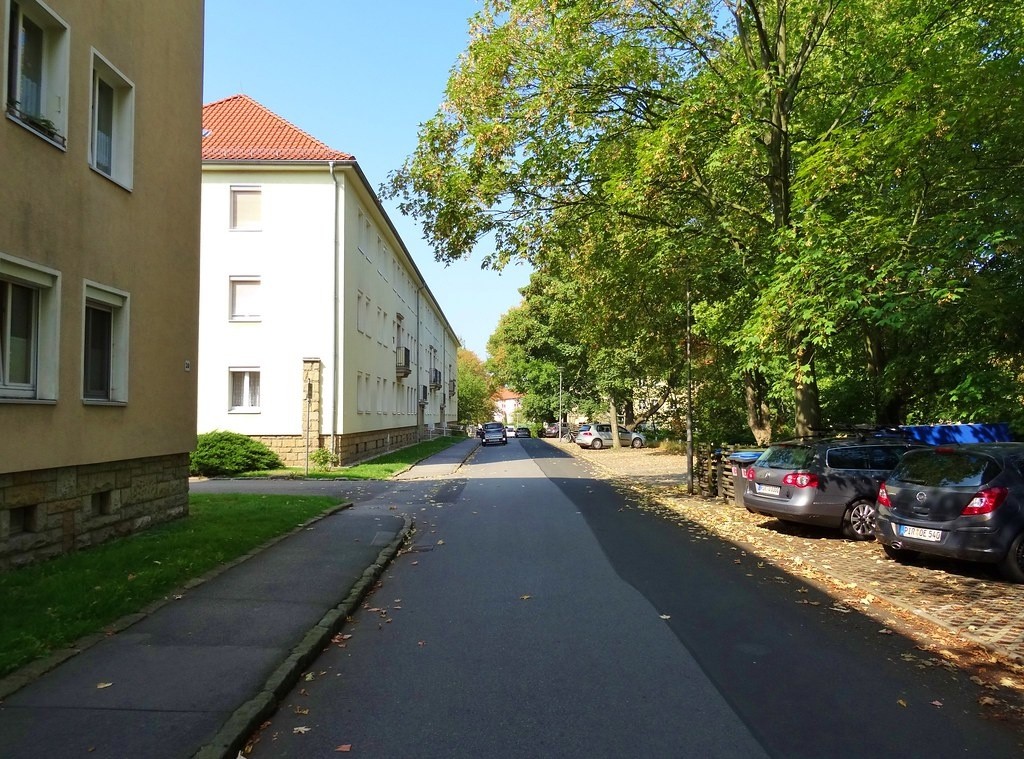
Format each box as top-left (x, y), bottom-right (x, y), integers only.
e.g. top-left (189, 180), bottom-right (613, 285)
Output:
top-left (481, 422), bottom-right (508, 447)
top-left (743, 435), bottom-right (932, 540)
top-left (875, 443), bottom-right (1024, 581)
top-left (576, 425), bottom-right (646, 449)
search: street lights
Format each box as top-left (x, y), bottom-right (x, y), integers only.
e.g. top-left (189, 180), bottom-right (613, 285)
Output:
top-left (557, 367), bottom-right (565, 442)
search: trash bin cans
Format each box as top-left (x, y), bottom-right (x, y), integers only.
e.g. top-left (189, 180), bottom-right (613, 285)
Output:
top-left (728, 452), bottom-right (764, 508)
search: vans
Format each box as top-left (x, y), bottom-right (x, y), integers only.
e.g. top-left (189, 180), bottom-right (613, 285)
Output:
top-left (546, 422), bottom-right (568, 437)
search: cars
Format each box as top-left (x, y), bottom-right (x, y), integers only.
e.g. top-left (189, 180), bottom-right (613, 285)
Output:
top-left (514, 427), bottom-right (531, 438)
top-left (505, 427), bottom-right (515, 438)
